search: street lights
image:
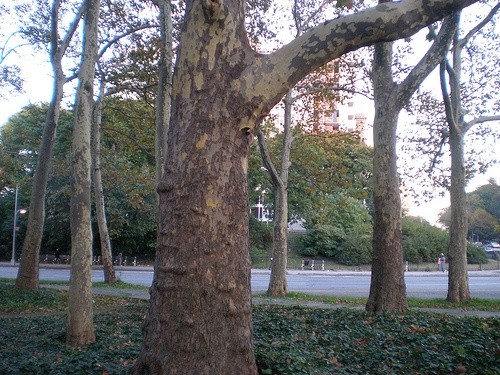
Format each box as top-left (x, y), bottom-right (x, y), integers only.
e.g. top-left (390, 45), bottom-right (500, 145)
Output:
top-left (11, 166), bottom-right (30, 266)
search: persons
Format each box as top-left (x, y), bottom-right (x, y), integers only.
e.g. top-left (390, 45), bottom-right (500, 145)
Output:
top-left (54, 248), bottom-right (60, 264)
top-left (116, 251), bottom-right (123, 267)
top-left (439, 253), bottom-right (447, 273)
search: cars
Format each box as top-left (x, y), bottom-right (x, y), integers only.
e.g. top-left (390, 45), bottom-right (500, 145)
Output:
top-left (475, 240), bottom-right (500, 256)
